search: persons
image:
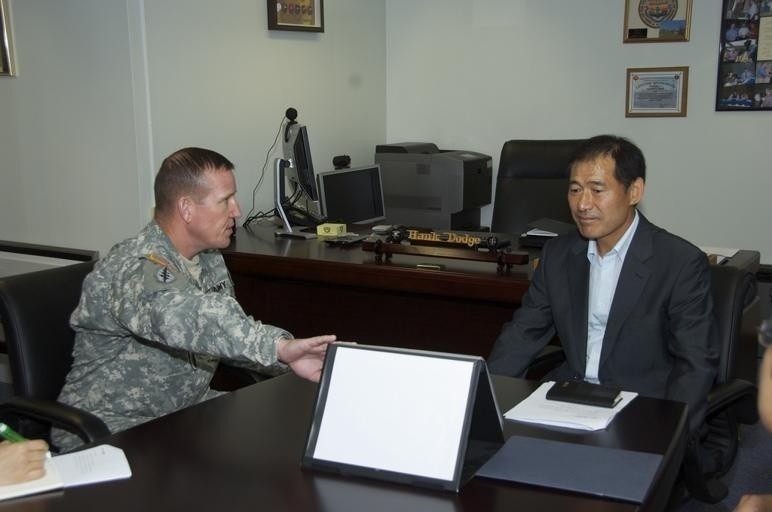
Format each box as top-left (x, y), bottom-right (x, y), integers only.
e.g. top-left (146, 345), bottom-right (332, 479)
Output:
top-left (720, 0), bottom-right (771, 108)
top-left (49, 147), bottom-right (357, 453)
top-left (484, 135), bottom-right (722, 439)
top-left (1, 435), bottom-right (50, 488)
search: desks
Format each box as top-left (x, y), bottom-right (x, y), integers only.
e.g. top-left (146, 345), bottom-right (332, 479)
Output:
top-left (210, 224), bottom-right (546, 394)
top-left (0, 372), bottom-right (689, 512)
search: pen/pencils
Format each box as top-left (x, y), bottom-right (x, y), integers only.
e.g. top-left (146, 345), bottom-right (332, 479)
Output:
top-left (0, 422), bottom-right (51, 461)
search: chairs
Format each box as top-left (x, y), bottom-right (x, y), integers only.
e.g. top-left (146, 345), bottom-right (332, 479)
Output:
top-left (491, 137), bottom-right (584, 235)
top-left (672, 250), bottom-right (761, 509)
top-left (0, 262), bottom-right (111, 449)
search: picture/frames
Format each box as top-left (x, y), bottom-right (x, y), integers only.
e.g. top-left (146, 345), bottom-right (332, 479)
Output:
top-left (268, 0), bottom-right (325, 33)
top-left (715, 0), bottom-right (772, 111)
top-left (622, 0), bottom-right (693, 44)
top-left (0, 0), bottom-right (18, 78)
top-left (625, 66), bottom-right (689, 118)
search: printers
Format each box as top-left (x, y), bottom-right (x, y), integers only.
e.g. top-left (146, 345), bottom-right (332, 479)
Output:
top-left (375, 142), bottom-right (493, 233)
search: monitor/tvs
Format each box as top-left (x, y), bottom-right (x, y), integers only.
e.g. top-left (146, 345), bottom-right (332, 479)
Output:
top-left (272, 121), bottom-right (322, 241)
top-left (317, 163), bottom-right (386, 231)
